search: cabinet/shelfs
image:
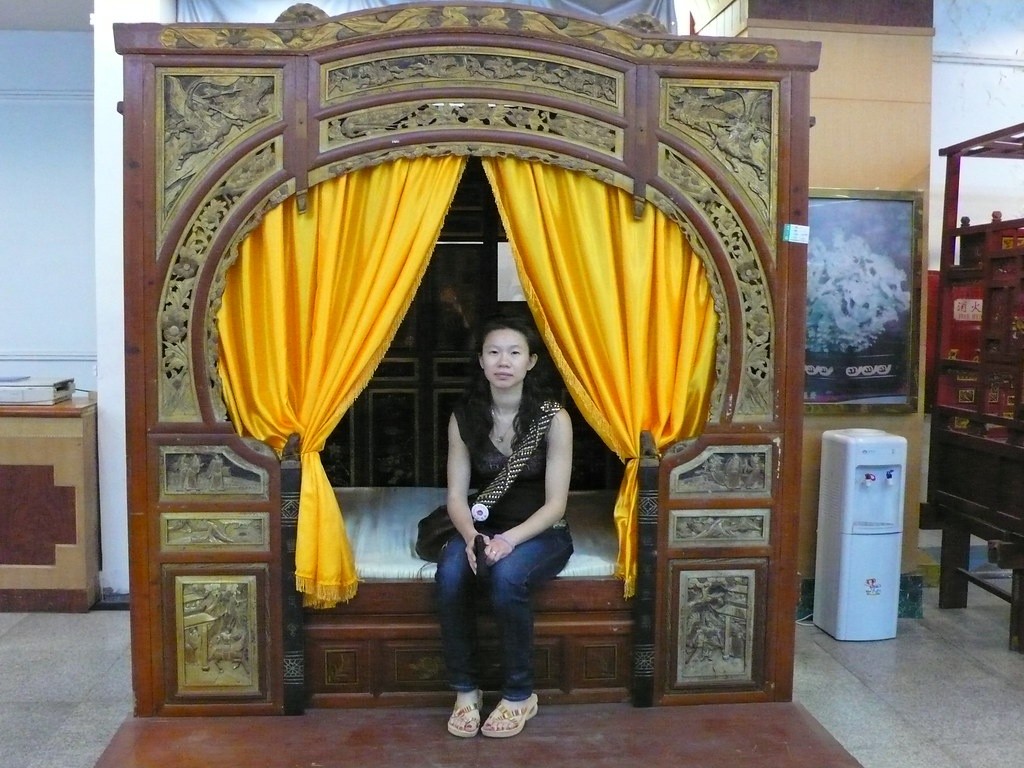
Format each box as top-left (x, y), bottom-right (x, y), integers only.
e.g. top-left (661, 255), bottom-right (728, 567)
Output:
top-left (0, 397), bottom-right (102, 613)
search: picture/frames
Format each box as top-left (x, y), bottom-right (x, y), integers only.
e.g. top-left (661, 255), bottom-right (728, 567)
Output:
top-left (802, 189), bottom-right (926, 415)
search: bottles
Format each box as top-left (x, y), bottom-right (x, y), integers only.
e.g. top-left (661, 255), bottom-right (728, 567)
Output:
top-left (472, 534), bottom-right (490, 585)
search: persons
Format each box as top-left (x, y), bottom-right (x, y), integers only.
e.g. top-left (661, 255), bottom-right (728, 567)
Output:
top-left (436, 318), bottom-right (573, 737)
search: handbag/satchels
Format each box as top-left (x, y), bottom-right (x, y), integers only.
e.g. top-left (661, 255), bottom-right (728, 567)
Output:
top-left (415, 492), bottom-right (478, 563)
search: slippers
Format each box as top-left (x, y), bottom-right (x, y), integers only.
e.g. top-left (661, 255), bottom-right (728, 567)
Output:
top-left (480, 691), bottom-right (538, 737)
top-left (447, 689), bottom-right (484, 738)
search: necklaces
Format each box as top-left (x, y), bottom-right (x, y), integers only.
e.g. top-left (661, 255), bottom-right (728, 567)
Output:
top-left (490, 415), bottom-right (514, 442)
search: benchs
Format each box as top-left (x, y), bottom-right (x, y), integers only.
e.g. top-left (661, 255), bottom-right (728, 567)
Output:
top-left (308, 480), bottom-right (629, 705)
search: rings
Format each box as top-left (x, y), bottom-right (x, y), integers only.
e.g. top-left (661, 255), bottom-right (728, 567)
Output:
top-left (491, 550), bottom-right (497, 554)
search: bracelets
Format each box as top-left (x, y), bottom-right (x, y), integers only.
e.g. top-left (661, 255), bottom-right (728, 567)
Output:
top-left (493, 534), bottom-right (515, 552)
